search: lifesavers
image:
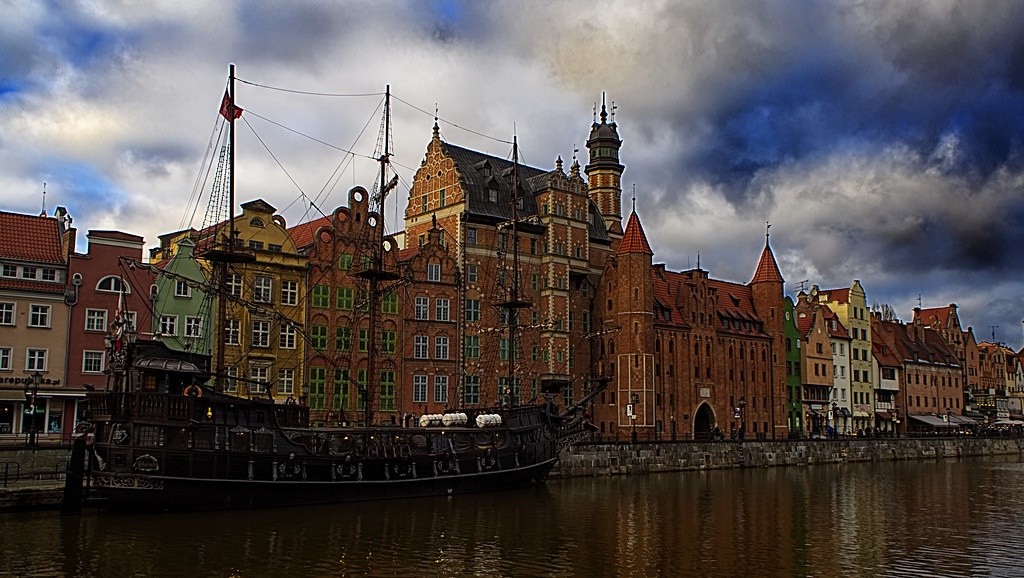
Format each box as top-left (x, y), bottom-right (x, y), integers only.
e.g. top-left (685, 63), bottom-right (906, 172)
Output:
top-left (183, 385), bottom-right (202, 398)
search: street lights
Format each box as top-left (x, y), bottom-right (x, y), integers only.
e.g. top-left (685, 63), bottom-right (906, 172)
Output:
top-left (629, 394), bottom-right (640, 446)
top-left (945, 407), bottom-right (951, 438)
top-left (831, 401), bottom-right (838, 440)
top-left (738, 395), bottom-right (745, 442)
top-left (23, 371), bottom-right (43, 446)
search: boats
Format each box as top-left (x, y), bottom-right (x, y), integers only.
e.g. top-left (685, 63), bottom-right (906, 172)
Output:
top-left (53, 63), bottom-right (612, 512)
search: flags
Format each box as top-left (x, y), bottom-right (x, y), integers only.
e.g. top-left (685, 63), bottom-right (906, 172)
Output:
top-left (575, 149), bottom-right (580, 152)
top-left (613, 106), bottom-right (617, 109)
top-left (219, 88), bottom-right (244, 123)
